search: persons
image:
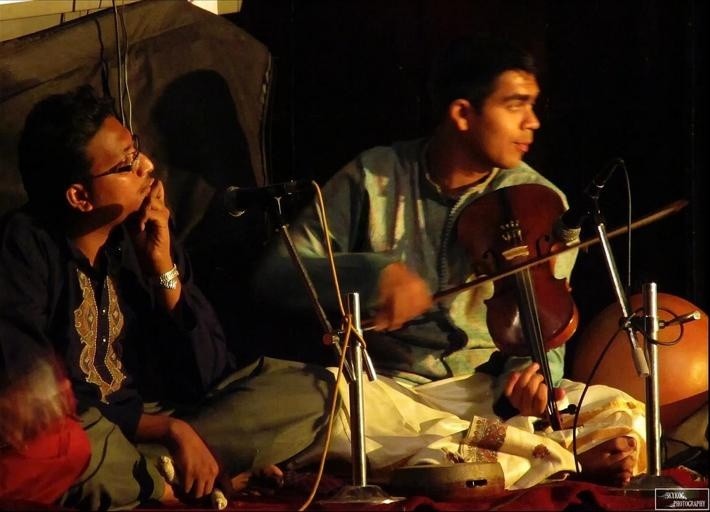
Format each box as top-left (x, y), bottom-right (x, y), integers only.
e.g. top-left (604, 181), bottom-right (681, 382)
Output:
top-left (0, 87), bottom-right (638, 511)
top-left (0, 313), bottom-right (91, 511)
top-left (252, 27), bottom-right (662, 485)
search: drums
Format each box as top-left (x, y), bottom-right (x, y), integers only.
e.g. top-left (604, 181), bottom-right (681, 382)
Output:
top-left (390, 462), bottom-right (504, 499)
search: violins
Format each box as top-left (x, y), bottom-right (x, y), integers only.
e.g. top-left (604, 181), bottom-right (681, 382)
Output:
top-left (454, 182), bottom-right (581, 434)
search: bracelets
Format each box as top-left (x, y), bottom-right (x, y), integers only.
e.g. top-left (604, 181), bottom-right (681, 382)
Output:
top-left (155, 262), bottom-right (180, 292)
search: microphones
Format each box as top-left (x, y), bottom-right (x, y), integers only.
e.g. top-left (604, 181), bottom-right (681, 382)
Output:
top-left (224, 179), bottom-right (298, 219)
top-left (554, 159), bottom-right (619, 243)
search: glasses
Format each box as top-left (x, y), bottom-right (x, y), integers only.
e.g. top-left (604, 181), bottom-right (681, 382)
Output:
top-left (78, 133), bottom-right (143, 185)
